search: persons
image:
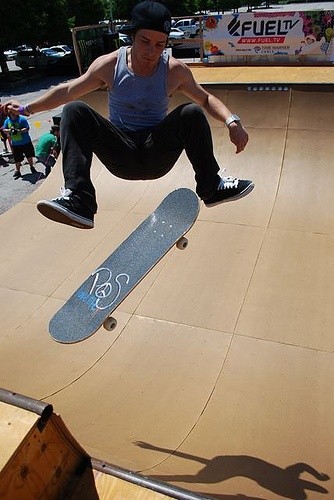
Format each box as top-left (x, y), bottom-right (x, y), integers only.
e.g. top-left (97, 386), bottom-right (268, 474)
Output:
top-left (34, 125), bottom-right (59, 178)
top-left (1, 109), bottom-right (36, 179)
top-left (0, 1), bottom-right (255, 229)
top-left (0, 98), bottom-right (9, 155)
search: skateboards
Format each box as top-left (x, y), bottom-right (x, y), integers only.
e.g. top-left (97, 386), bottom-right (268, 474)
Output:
top-left (48, 187), bottom-right (200, 345)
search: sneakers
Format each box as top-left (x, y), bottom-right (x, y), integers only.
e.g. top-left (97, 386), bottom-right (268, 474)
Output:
top-left (202, 170), bottom-right (255, 207)
top-left (36, 186), bottom-right (95, 230)
top-left (31, 167), bottom-right (38, 176)
top-left (14, 171), bottom-right (21, 177)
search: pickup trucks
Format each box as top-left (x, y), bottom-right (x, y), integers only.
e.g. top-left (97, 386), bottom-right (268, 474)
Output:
top-left (171, 19), bottom-right (203, 35)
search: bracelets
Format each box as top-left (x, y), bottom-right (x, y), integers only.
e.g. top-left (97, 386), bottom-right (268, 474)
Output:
top-left (16, 104), bottom-right (33, 116)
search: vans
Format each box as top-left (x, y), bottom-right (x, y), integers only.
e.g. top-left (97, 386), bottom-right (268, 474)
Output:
top-left (16, 48), bottom-right (63, 70)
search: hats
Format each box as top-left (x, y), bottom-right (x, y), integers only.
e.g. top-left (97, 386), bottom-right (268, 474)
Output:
top-left (51, 125), bottom-right (60, 130)
top-left (117, 1), bottom-right (171, 35)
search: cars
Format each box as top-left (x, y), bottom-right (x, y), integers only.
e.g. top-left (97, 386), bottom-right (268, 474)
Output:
top-left (118, 32), bottom-right (132, 46)
top-left (3, 49), bottom-right (18, 61)
top-left (50, 45), bottom-right (72, 56)
top-left (168, 28), bottom-right (185, 47)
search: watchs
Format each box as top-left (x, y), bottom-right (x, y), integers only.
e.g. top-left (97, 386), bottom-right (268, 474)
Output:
top-left (224, 111), bottom-right (241, 126)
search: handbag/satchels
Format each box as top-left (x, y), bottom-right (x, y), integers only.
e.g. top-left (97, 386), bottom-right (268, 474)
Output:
top-left (0, 130), bottom-right (7, 140)
